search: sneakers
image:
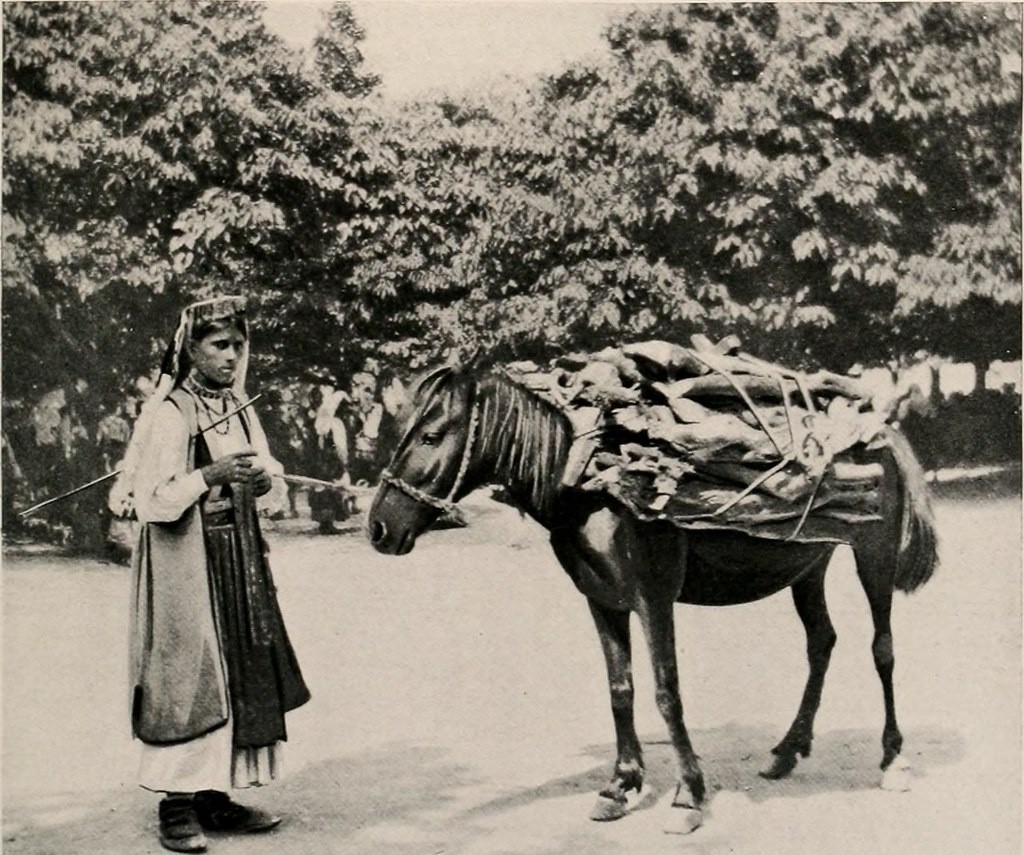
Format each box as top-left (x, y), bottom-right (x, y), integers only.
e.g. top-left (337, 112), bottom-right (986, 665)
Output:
top-left (192, 788), bottom-right (282, 833)
top-left (157, 795), bottom-right (209, 851)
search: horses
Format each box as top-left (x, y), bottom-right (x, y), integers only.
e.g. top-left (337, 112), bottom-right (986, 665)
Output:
top-left (367, 338), bottom-right (939, 833)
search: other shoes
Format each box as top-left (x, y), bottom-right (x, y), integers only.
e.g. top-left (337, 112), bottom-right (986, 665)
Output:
top-left (319, 520), bottom-right (340, 534)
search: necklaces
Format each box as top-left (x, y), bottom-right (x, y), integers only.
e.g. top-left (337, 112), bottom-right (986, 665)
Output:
top-left (198, 391), bottom-right (230, 435)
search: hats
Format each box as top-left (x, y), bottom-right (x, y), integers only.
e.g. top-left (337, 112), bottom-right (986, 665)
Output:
top-left (183, 281), bottom-right (248, 326)
top-left (314, 390), bottom-right (351, 435)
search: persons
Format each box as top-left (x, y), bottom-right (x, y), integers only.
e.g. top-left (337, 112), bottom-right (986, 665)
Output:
top-left (109, 298), bottom-right (311, 852)
top-left (303, 373), bottom-right (383, 534)
